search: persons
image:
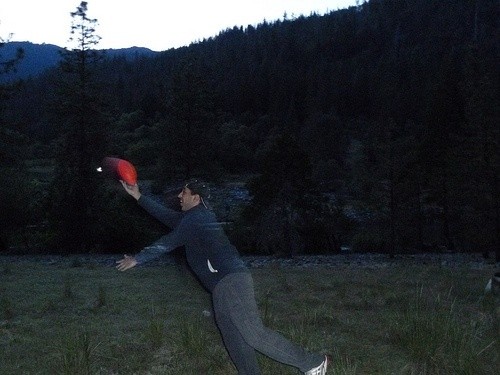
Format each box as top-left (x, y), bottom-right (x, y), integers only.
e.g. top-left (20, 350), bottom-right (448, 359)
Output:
top-left (116, 180), bottom-right (327, 375)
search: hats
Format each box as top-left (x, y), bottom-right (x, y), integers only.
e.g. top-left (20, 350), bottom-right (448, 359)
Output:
top-left (182, 176), bottom-right (213, 211)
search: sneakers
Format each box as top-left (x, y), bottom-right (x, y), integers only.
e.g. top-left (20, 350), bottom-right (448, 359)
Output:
top-left (304, 355), bottom-right (328, 375)
